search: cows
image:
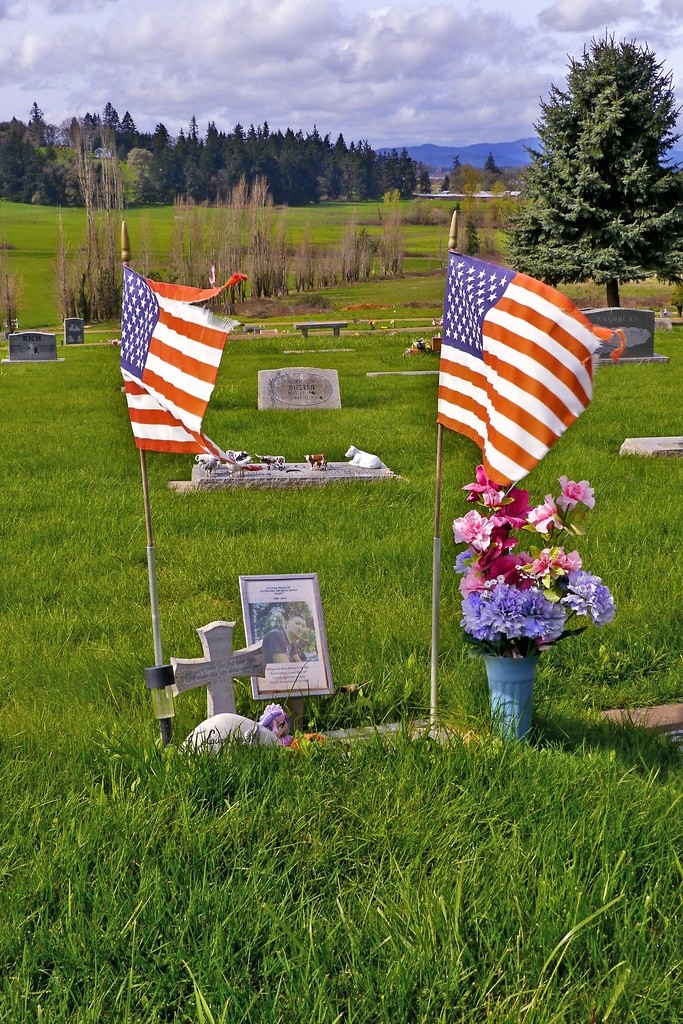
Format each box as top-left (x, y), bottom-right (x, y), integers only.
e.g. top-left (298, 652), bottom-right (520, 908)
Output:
top-left (195, 449), bottom-right (286, 477)
top-left (303, 454), bottom-right (328, 471)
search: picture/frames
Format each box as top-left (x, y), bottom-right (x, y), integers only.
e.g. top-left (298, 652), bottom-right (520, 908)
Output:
top-left (239, 572), bottom-right (334, 702)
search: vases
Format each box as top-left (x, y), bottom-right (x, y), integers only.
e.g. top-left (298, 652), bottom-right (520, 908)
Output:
top-left (485, 657), bottom-right (535, 745)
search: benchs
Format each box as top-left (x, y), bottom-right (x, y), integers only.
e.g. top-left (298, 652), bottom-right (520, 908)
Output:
top-left (294, 322), bottom-right (348, 338)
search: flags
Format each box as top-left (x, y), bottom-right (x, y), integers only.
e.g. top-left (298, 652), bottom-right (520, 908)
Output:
top-left (118, 266), bottom-right (249, 465)
top-left (435, 248), bottom-right (626, 486)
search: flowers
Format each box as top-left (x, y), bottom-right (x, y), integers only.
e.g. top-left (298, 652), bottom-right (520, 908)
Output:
top-left (452, 465), bottom-right (616, 658)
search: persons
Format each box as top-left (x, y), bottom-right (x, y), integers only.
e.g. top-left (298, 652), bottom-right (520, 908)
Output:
top-left (263, 613), bottom-right (306, 664)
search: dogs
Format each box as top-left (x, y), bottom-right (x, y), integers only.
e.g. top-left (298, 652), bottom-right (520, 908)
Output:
top-left (344, 445), bottom-right (382, 469)
